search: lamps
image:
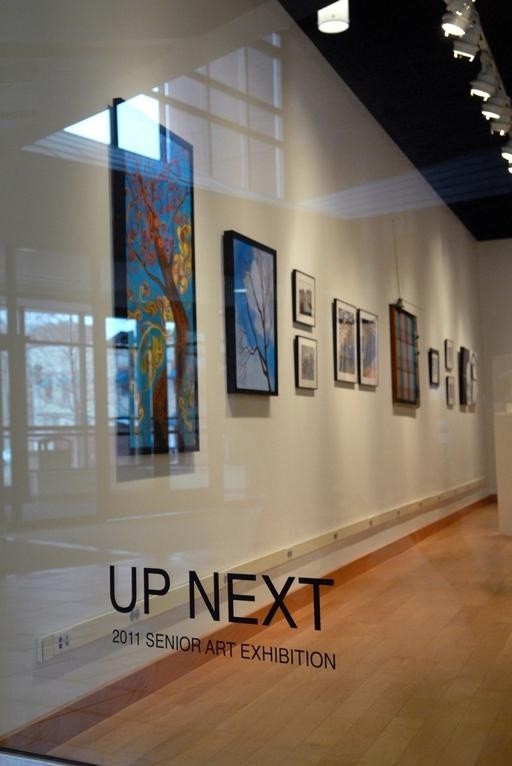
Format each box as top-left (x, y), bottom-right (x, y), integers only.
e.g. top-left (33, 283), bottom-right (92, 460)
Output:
top-left (439, 0), bottom-right (511, 176)
top-left (316, 0), bottom-right (350, 36)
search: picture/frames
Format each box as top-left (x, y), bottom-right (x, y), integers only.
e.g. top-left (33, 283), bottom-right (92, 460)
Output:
top-left (293, 335), bottom-right (320, 391)
top-left (332, 296), bottom-right (358, 384)
top-left (111, 94), bottom-right (203, 456)
top-left (290, 268), bottom-right (317, 329)
top-left (428, 339), bottom-right (456, 407)
top-left (388, 301), bottom-right (419, 409)
top-left (358, 307), bottom-right (380, 388)
top-left (222, 229), bottom-right (282, 399)
